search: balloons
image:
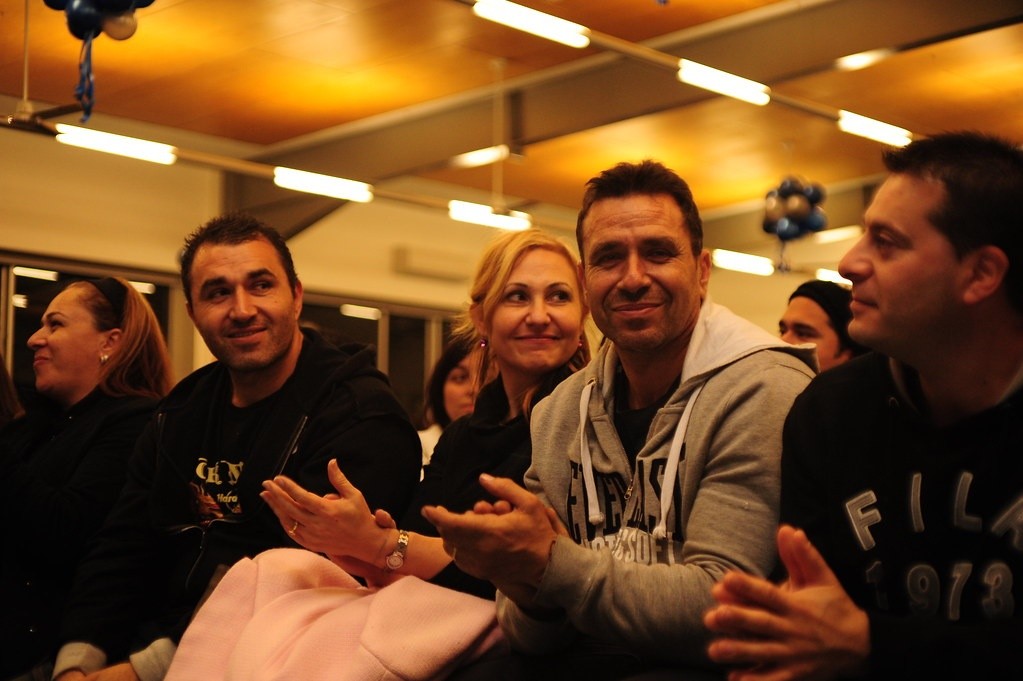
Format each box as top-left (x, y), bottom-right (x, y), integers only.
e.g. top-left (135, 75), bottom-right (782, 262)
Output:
top-left (761, 176), bottom-right (827, 243)
top-left (39, 0), bottom-right (156, 41)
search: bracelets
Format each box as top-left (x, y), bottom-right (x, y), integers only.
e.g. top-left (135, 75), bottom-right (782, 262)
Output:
top-left (370, 528), bottom-right (389, 566)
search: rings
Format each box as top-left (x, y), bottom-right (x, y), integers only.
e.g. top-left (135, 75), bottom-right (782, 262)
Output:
top-left (288, 522), bottom-right (297, 537)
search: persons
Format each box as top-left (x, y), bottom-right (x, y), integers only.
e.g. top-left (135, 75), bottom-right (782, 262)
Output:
top-left (0, 211), bottom-right (422, 681)
top-left (776, 280), bottom-right (861, 372)
top-left (262, 164), bottom-right (817, 681)
top-left (706, 130), bottom-right (1023, 681)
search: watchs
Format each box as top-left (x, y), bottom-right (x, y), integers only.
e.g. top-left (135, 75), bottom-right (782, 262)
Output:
top-left (382, 529), bottom-right (409, 573)
